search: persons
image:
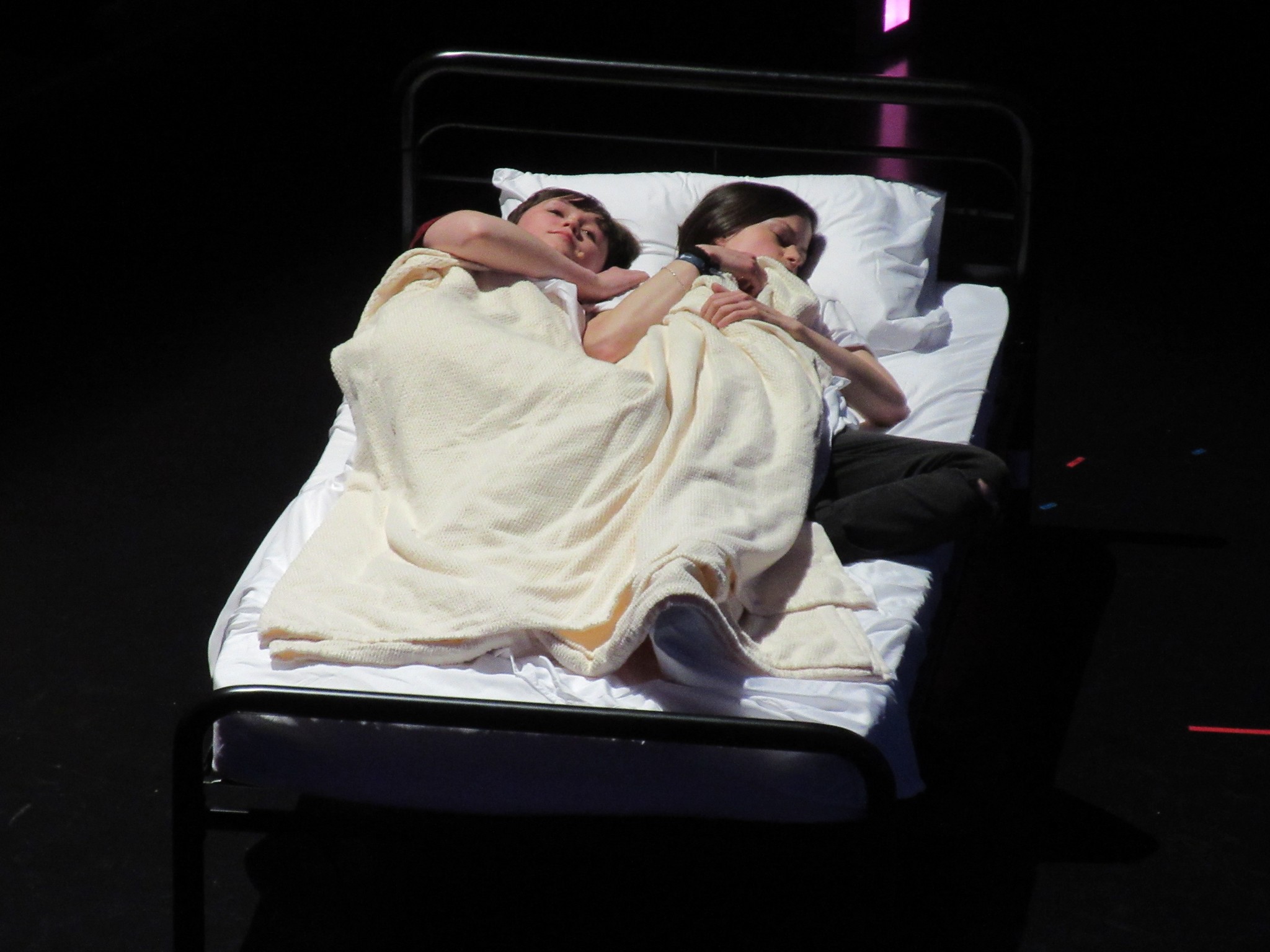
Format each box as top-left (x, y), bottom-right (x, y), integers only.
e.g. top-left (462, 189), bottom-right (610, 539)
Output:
top-left (407, 189), bottom-right (655, 299)
top-left (577, 176), bottom-right (1004, 566)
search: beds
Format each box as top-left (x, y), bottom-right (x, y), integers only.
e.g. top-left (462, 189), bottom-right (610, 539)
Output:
top-left (173, 46), bottom-right (1043, 952)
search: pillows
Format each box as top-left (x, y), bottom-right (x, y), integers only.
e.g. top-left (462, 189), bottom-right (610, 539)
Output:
top-left (486, 166), bottom-right (949, 354)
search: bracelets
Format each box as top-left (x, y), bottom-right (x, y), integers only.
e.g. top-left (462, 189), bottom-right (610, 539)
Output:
top-left (674, 243), bottom-right (722, 279)
top-left (660, 266), bottom-right (691, 293)
